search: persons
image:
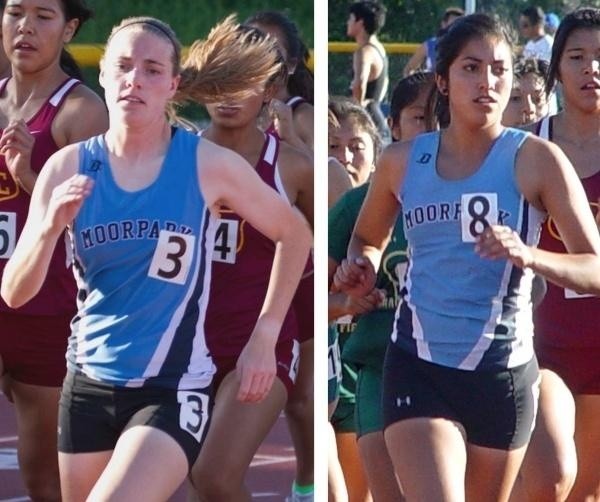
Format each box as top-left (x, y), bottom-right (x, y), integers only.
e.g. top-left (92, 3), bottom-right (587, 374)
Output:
top-left (328, 0), bottom-right (600, 502)
top-left (0, 0), bottom-right (313, 502)
